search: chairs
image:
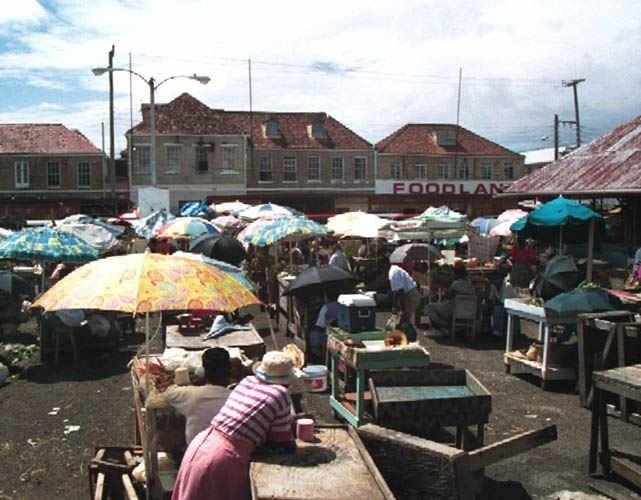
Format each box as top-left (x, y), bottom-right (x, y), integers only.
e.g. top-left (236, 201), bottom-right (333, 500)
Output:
top-left (452, 294), bottom-right (480, 343)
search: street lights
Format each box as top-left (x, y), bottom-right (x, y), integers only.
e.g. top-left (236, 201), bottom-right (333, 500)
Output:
top-left (90, 67), bottom-right (212, 185)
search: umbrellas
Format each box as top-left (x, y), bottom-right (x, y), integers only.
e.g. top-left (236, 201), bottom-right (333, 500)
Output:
top-left (541, 282), bottom-right (625, 327)
top-left (21, 250), bottom-right (269, 342)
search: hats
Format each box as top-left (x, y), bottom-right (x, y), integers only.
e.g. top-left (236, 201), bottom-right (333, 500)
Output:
top-left (251, 350), bottom-right (296, 384)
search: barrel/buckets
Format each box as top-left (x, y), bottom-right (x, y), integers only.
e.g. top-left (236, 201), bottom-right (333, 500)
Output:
top-left (302, 364), bottom-right (328, 394)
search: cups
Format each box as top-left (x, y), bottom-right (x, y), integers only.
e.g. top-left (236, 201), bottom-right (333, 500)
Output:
top-left (295, 419), bottom-right (313, 441)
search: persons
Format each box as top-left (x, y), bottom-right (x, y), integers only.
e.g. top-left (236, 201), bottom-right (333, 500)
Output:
top-left (374, 257), bottom-right (421, 342)
top-left (139, 346), bottom-right (241, 463)
top-left (424, 266), bottom-right (475, 339)
top-left (165, 348), bottom-right (301, 500)
top-left (304, 287), bottom-right (341, 364)
top-left (478, 269), bottom-right (524, 340)
